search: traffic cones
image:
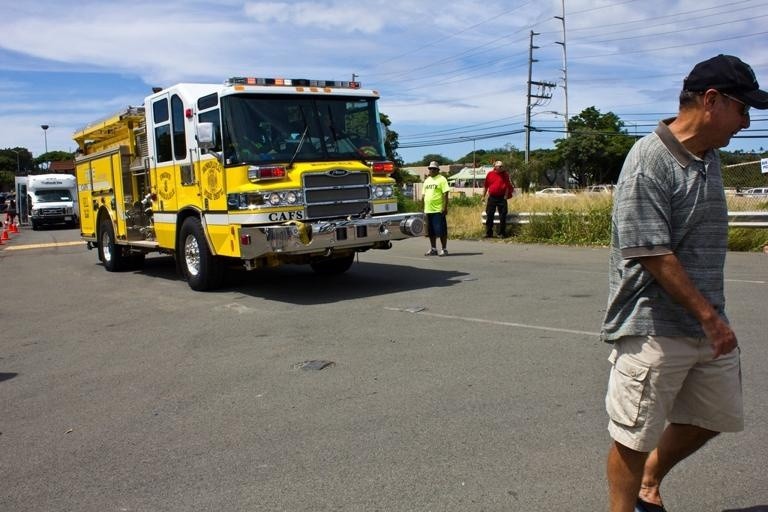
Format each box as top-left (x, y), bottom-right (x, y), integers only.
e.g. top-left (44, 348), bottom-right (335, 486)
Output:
top-left (6, 215), bottom-right (13, 230)
top-left (0, 234), bottom-right (5, 245)
top-left (11, 220), bottom-right (19, 233)
top-left (2, 223), bottom-right (11, 240)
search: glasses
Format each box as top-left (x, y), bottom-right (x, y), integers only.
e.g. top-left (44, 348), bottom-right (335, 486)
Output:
top-left (699, 89), bottom-right (752, 116)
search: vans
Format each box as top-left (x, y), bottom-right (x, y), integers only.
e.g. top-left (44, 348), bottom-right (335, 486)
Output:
top-left (742, 188), bottom-right (768, 197)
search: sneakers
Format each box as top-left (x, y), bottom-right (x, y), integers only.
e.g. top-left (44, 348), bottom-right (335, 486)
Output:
top-left (424, 248), bottom-right (437, 256)
top-left (438, 249), bottom-right (448, 256)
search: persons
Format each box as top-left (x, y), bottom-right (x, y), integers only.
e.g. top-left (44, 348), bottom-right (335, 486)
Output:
top-left (482, 161), bottom-right (513, 238)
top-left (421, 161), bottom-right (449, 257)
top-left (8, 200), bottom-right (16, 223)
top-left (599, 55), bottom-right (767, 511)
top-left (319, 112), bottom-right (337, 137)
top-left (239, 128), bottom-right (277, 162)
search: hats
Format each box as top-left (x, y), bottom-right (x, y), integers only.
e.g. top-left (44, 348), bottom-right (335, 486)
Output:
top-left (684, 54), bottom-right (768, 109)
top-left (427, 161), bottom-right (439, 170)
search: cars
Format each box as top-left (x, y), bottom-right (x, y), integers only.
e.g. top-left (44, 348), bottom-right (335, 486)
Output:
top-left (0, 192), bottom-right (15, 213)
top-left (401, 186), bottom-right (412, 199)
top-left (583, 183), bottom-right (617, 194)
top-left (536, 185), bottom-right (576, 197)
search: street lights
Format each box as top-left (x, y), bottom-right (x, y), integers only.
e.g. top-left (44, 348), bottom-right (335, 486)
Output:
top-left (457, 135), bottom-right (476, 196)
top-left (10, 149), bottom-right (25, 174)
top-left (41, 123), bottom-right (51, 172)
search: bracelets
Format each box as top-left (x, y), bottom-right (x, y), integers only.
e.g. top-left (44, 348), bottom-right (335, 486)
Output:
top-left (504, 194), bottom-right (508, 196)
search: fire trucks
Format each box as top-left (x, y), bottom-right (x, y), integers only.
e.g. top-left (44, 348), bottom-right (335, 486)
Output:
top-left (74, 68), bottom-right (432, 292)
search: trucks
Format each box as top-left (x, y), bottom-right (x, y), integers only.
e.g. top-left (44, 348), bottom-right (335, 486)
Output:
top-left (15, 172), bottom-right (79, 230)
